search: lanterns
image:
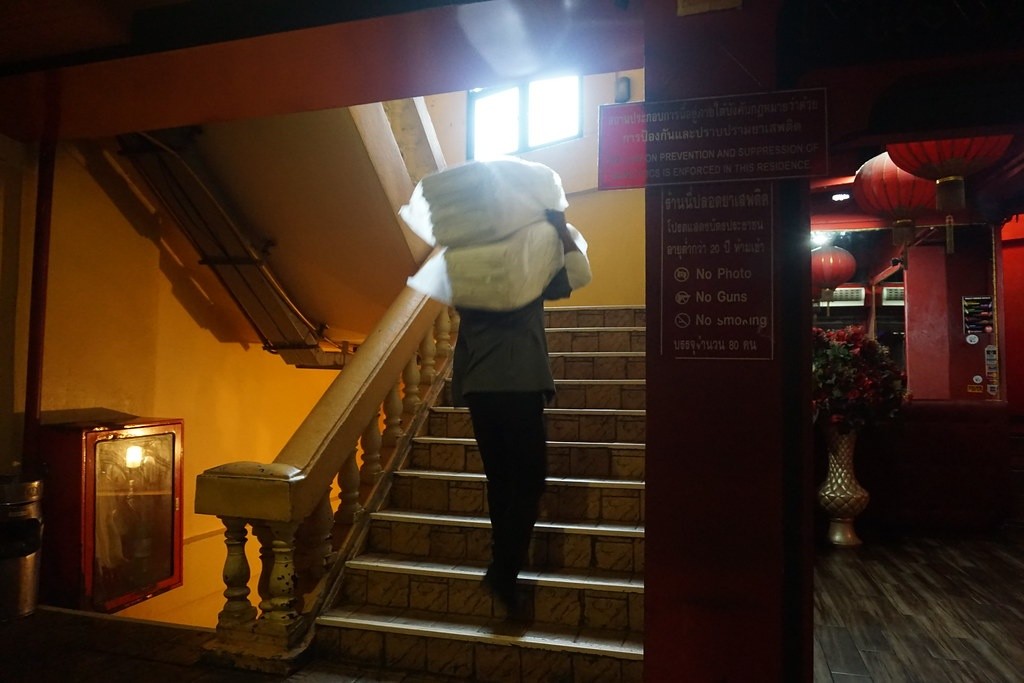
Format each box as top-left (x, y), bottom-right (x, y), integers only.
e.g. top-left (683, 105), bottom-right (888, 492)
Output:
top-left (810, 135), bottom-right (1016, 316)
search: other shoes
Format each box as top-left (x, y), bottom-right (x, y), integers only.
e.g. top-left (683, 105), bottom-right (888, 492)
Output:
top-left (485, 572), bottom-right (531, 624)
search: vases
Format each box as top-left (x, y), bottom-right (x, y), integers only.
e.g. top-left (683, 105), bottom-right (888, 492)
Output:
top-left (816, 404), bottom-right (871, 546)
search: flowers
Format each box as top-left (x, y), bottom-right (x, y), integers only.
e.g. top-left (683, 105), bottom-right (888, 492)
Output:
top-left (809, 323), bottom-right (924, 426)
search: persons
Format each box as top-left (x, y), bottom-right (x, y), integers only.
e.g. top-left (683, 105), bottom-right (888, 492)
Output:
top-left (456, 206), bottom-right (593, 622)
